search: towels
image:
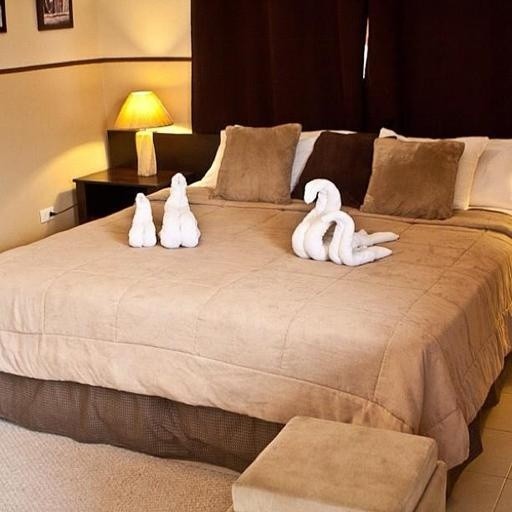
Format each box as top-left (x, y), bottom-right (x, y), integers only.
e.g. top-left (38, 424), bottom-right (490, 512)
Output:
top-left (291, 179), bottom-right (399, 261)
top-left (320, 210), bottom-right (392, 266)
top-left (158, 173), bottom-right (201, 248)
top-left (128, 193), bottom-right (157, 247)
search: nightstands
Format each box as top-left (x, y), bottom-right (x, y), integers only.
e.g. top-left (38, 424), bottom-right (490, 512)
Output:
top-left (73, 159), bottom-right (194, 225)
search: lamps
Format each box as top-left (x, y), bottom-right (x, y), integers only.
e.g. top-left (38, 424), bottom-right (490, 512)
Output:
top-left (113, 91), bottom-right (174, 176)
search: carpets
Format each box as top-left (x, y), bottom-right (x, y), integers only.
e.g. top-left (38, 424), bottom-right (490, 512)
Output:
top-left (1, 419), bottom-right (242, 511)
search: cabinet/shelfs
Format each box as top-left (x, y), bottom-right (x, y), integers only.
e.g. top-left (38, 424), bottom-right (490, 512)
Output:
top-left (191, 1), bottom-right (512, 138)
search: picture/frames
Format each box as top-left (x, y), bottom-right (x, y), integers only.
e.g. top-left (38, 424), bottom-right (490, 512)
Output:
top-left (36, 0), bottom-right (73, 31)
top-left (0, 0), bottom-right (7, 33)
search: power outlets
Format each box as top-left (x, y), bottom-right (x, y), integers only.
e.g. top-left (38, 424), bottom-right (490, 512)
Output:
top-left (40, 207), bottom-right (54, 223)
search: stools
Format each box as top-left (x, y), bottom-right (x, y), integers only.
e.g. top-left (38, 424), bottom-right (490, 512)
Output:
top-left (227, 415), bottom-right (448, 511)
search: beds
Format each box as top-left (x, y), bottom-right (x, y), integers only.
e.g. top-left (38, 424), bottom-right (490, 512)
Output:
top-left (0, 123), bottom-right (512, 474)
